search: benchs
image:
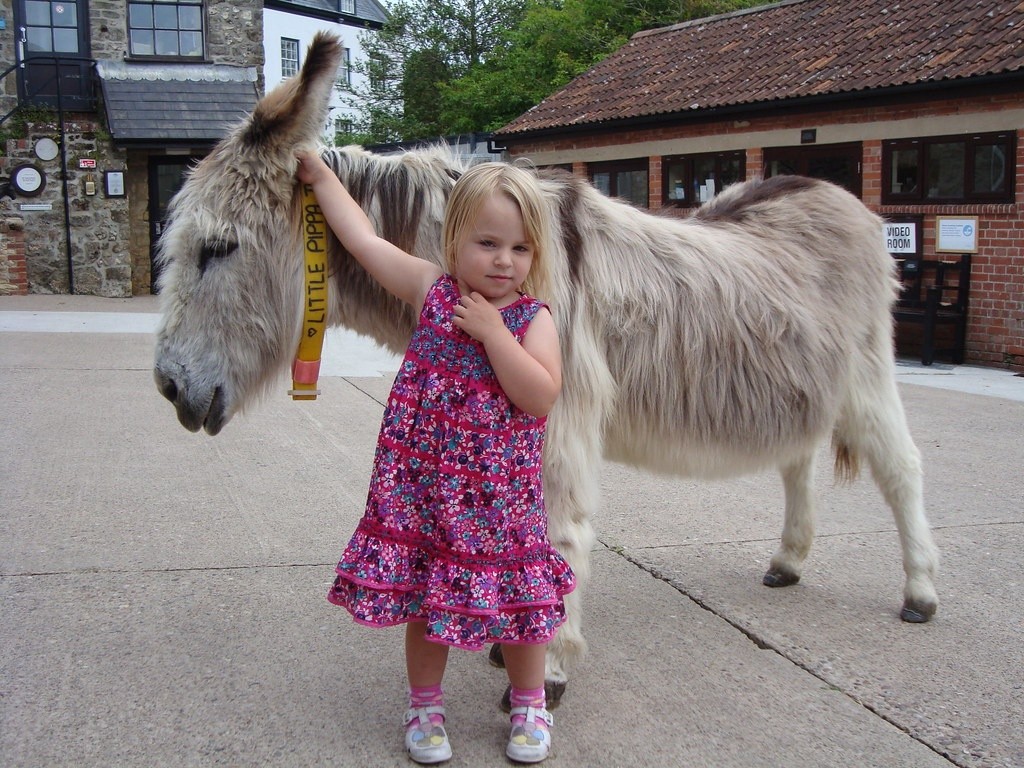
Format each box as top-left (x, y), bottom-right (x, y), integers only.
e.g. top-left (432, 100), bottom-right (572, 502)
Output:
top-left (893, 252), bottom-right (971, 365)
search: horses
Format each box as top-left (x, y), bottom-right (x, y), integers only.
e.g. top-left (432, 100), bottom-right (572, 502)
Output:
top-left (154, 31), bottom-right (938, 712)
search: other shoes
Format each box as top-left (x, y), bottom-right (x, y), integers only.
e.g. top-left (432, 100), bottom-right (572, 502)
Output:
top-left (402, 705), bottom-right (453, 764)
top-left (506, 705), bottom-right (554, 764)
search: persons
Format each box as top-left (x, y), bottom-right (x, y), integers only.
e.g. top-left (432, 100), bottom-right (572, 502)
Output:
top-left (292, 150), bottom-right (578, 763)
